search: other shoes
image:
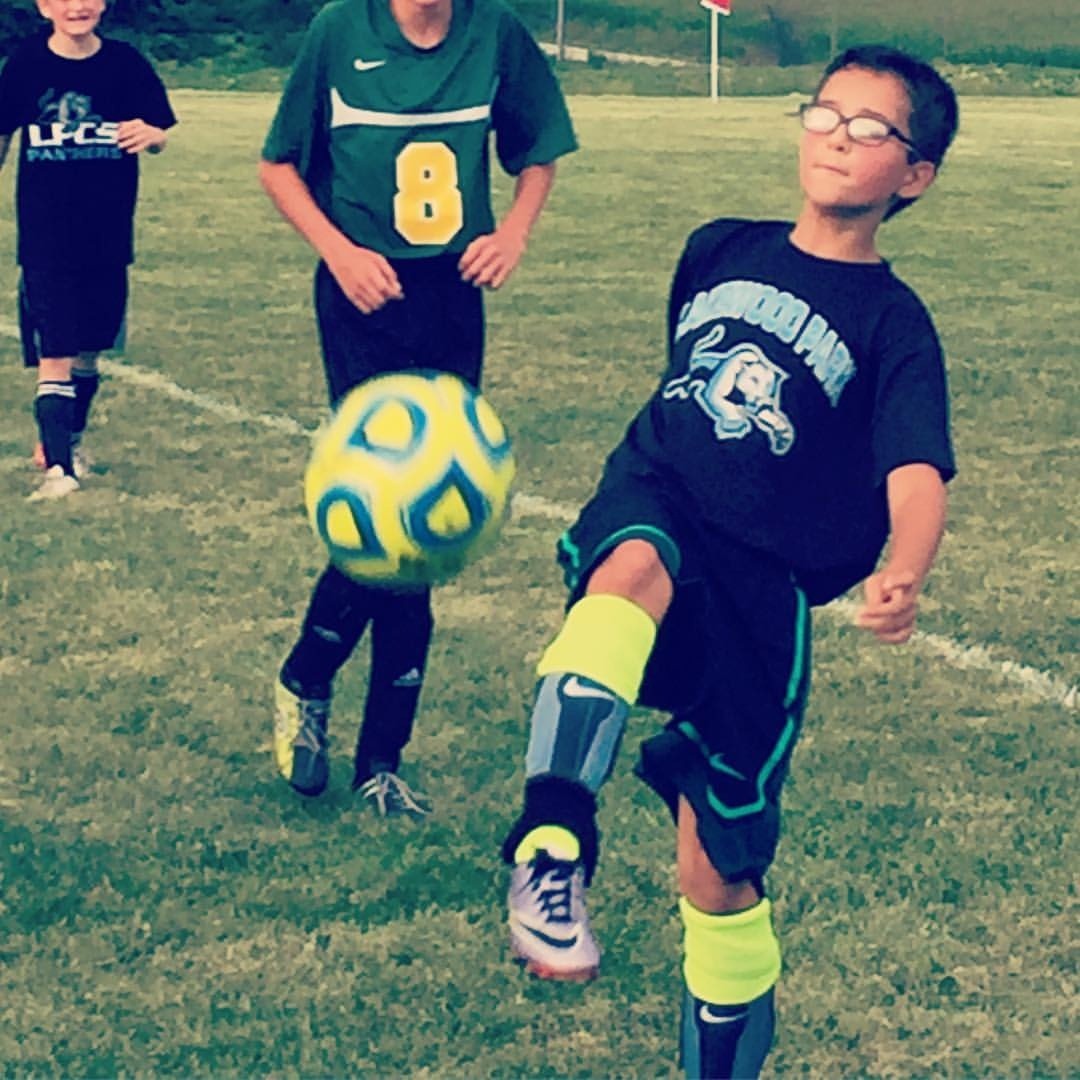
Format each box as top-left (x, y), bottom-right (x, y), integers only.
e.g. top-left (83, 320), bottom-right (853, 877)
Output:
top-left (35, 440), bottom-right (87, 480)
top-left (26, 462), bottom-right (79, 503)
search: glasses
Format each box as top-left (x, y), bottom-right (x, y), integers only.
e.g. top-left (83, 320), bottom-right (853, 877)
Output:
top-left (800, 102), bottom-right (926, 161)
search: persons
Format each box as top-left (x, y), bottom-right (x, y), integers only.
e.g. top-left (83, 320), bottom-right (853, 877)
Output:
top-left (262, 0), bottom-right (578, 821)
top-left (1, 0), bottom-right (178, 499)
top-left (498, 44), bottom-right (960, 1080)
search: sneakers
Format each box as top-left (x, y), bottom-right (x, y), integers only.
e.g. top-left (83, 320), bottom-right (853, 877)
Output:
top-left (272, 667), bottom-right (331, 796)
top-left (504, 846), bottom-right (599, 984)
top-left (357, 773), bottom-right (431, 824)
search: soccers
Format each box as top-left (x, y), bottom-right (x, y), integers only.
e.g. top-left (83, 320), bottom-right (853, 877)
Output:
top-left (304, 369), bottom-right (515, 589)
